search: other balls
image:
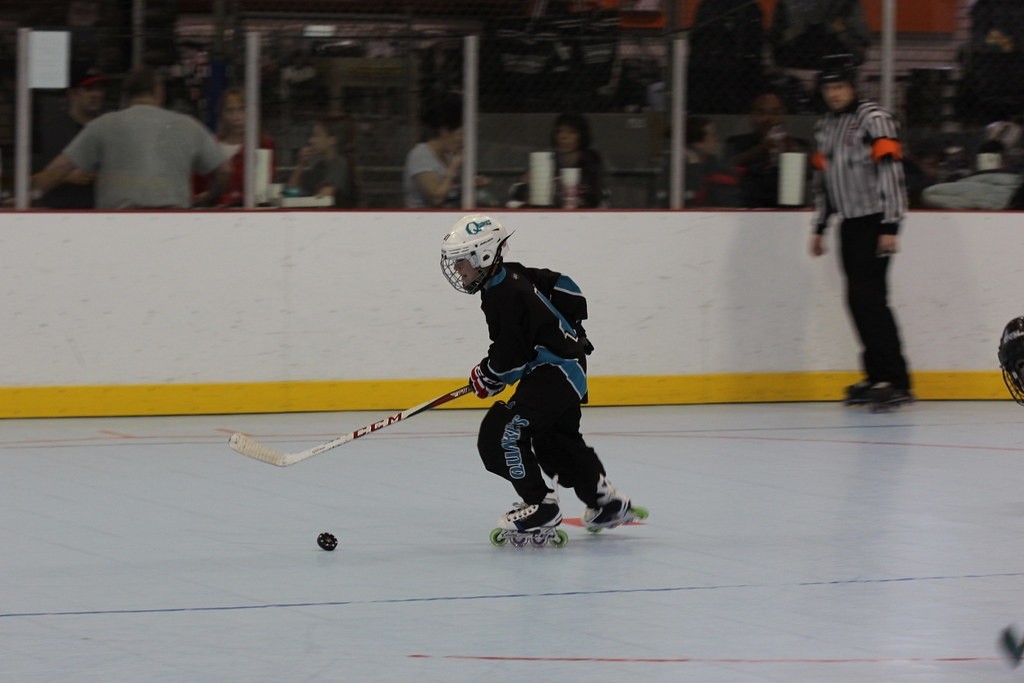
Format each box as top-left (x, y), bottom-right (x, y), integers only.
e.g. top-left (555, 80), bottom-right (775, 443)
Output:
top-left (317, 532), bottom-right (338, 551)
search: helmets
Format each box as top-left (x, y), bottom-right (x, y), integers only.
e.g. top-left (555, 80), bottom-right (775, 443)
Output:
top-left (997, 316), bottom-right (1024, 406)
top-left (440, 214), bottom-right (510, 294)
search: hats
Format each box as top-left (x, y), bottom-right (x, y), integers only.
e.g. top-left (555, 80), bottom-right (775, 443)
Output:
top-left (815, 53), bottom-right (859, 80)
top-left (71, 61), bottom-right (111, 88)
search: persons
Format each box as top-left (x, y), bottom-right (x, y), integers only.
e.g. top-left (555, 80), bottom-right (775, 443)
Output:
top-left (663, 0), bottom-right (1024, 211)
top-left (2, 60), bottom-right (353, 209)
top-left (998, 316), bottom-right (1024, 406)
top-left (440, 214), bottom-right (631, 531)
top-left (808, 57), bottom-right (911, 404)
top-left (405, 93), bottom-right (463, 210)
top-left (509, 110), bottom-right (605, 209)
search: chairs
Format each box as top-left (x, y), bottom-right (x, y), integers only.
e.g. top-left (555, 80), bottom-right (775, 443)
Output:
top-left (349, 163), bottom-right (377, 209)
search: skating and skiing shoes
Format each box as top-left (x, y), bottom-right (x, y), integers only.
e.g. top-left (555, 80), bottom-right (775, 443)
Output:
top-left (585, 473), bottom-right (648, 534)
top-left (490, 474), bottom-right (569, 548)
top-left (846, 377), bottom-right (913, 413)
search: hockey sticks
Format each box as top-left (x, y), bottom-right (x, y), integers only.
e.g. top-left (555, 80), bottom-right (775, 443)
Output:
top-left (227, 385), bottom-right (475, 470)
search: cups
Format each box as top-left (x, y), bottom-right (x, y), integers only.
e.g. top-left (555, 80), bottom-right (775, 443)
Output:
top-left (560, 168), bottom-right (581, 208)
top-left (267, 183), bottom-right (285, 207)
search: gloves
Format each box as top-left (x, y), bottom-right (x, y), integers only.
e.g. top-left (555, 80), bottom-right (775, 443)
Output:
top-left (468, 357), bottom-right (507, 399)
